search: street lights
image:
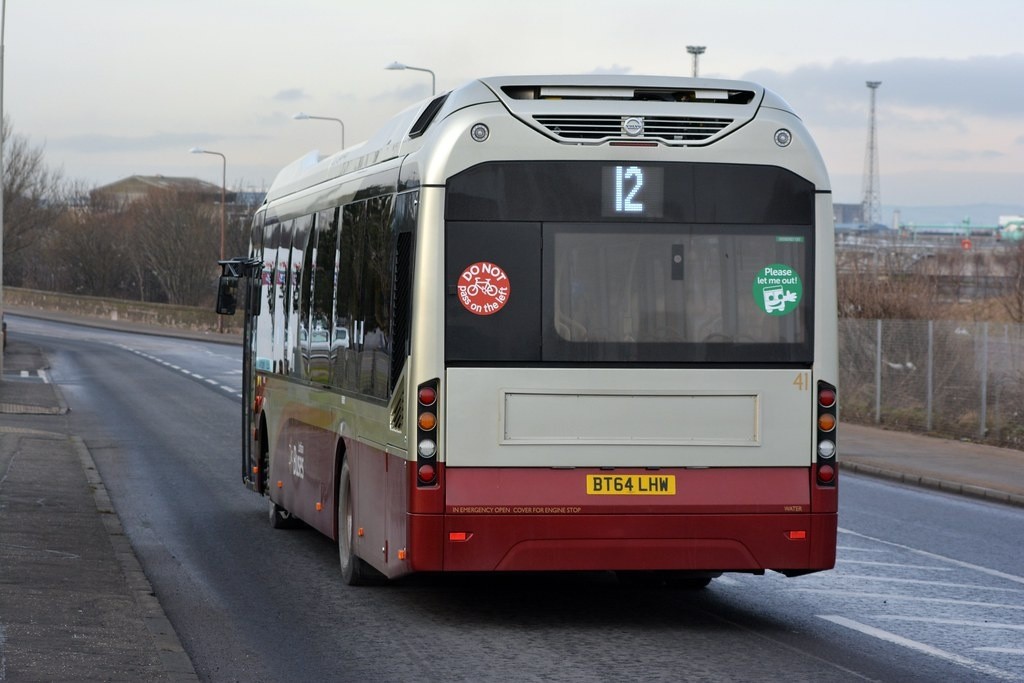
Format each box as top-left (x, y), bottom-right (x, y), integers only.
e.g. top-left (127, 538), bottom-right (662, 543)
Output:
top-left (292, 111), bottom-right (345, 151)
top-left (686, 45), bottom-right (707, 78)
top-left (383, 62), bottom-right (436, 97)
top-left (189, 147), bottom-right (227, 334)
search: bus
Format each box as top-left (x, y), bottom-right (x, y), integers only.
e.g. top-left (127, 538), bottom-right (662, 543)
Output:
top-left (214, 75), bottom-right (842, 588)
